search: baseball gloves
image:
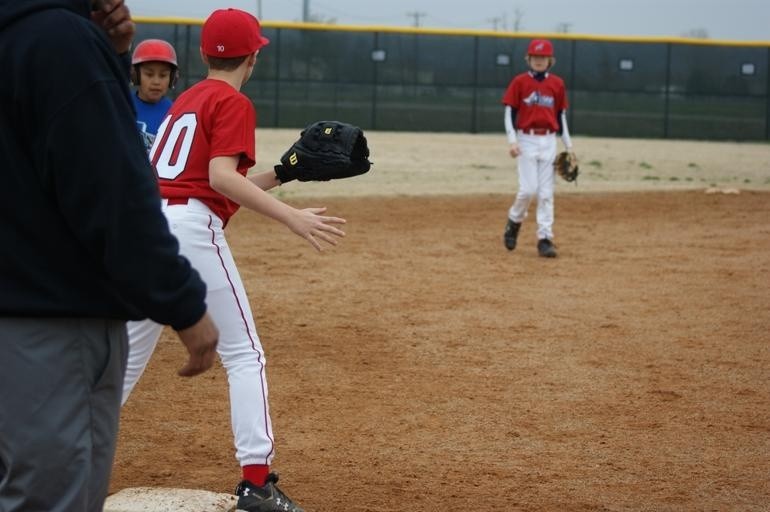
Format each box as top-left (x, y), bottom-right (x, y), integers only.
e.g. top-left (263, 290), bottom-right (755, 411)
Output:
top-left (274, 121), bottom-right (370, 183)
top-left (553, 152), bottom-right (580, 182)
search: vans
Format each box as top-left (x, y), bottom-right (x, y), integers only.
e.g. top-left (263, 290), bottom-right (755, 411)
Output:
top-left (660, 83), bottom-right (685, 101)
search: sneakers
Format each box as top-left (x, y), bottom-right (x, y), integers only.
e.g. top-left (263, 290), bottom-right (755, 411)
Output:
top-left (502, 216), bottom-right (523, 251)
top-left (536, 238), bottom-right (557, 258)
top-left (233, 470), bottom-right (308, 512)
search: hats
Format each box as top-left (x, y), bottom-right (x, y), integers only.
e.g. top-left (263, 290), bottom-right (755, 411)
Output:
top-left (526, 38), bottom-right (554, 57)
top-left (200, 7), bottom-right (271, 61)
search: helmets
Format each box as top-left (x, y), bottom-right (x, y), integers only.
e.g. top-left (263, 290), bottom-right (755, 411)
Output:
top-left (128, 39), bottom-right (183, 90)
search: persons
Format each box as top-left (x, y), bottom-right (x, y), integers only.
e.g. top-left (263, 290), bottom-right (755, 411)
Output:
top-left (0, 0), bottom-right (219, 511)
top-left (117, 8), bottom-right (372, 512)
top-left (129, 38), bottom-right (178, 157)
top-left (500, 39), bottom-right (578, 257)
